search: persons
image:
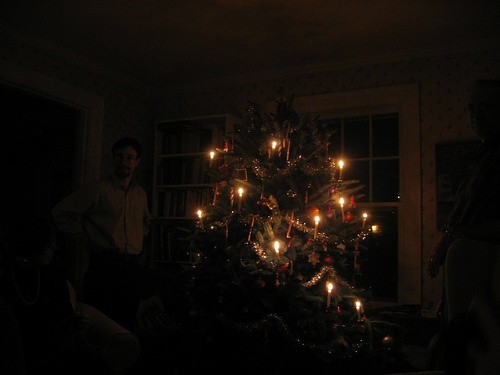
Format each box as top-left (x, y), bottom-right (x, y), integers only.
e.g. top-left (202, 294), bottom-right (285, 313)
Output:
top-left (57, 136), bottom-right (150, 332)
top-left (429, 79), bottom-right (500, 375)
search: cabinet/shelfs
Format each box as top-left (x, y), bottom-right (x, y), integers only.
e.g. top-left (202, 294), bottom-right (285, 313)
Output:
top-left (149, 114), bottom-right (240, 268)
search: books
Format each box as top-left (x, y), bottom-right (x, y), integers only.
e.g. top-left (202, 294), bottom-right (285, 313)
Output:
top-left (154, 123), bottom-right (225, 262)
